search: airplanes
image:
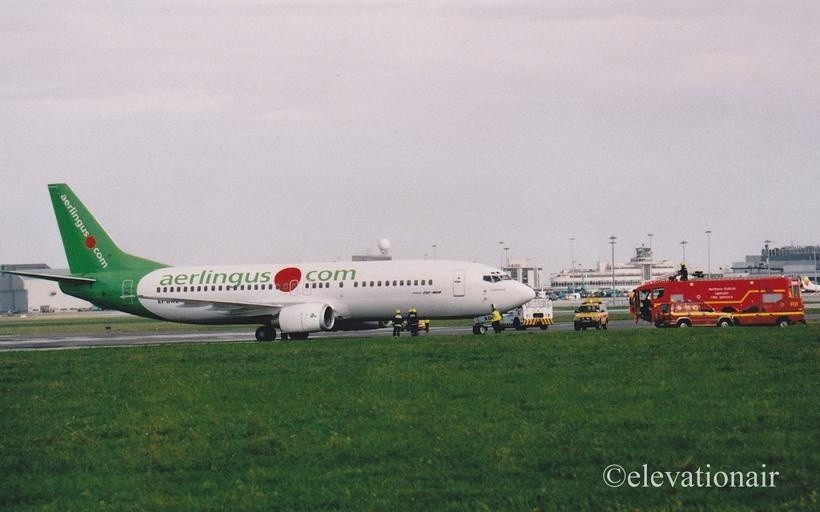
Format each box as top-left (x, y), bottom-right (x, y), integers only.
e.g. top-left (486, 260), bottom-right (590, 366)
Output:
top-left (0, 183), bottom-right (538, 343)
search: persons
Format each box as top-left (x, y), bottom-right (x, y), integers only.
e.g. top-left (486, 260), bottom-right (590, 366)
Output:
top-left (674, 262), bottom-right (689, 281)
top-left (407, 308), bottom-right (418, 336)
top-left (391, 308), bottom-right (404, 338)
top-left (490, 307), bottom-right (503, 334)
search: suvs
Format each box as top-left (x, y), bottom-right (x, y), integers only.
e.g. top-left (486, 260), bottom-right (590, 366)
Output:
top-left (572, 297), bottom-right (610, 331)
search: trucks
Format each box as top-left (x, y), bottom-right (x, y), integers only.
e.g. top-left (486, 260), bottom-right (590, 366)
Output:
top-left (472, 296), bottom-right (554, 332)
top-left (627, 273), bottom-right (807, 328)
